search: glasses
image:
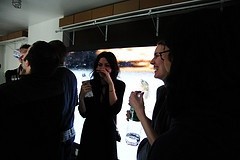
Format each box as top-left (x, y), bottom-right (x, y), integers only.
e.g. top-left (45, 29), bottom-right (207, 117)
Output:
top-left (155, 50), bottom-right (170, 58)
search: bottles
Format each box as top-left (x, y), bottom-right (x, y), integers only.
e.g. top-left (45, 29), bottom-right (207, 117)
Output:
top-left (6, 44), bottom-right (21, 57)
top-left (124, 91), bottom-right (144, 146)
top-left (82, 73), bottom-right (95, 97)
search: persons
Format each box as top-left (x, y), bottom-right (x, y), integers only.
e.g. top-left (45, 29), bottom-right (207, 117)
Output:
top-left (0, 40), bottom-right (78, 160)
top-left (78, 51), bottom-right (125, 160)
top-left (126, 39), bottom-right (194, 160)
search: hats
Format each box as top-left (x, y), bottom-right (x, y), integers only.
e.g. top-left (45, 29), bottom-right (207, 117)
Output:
top-left (159, 35), bottom-right (194, 70)
